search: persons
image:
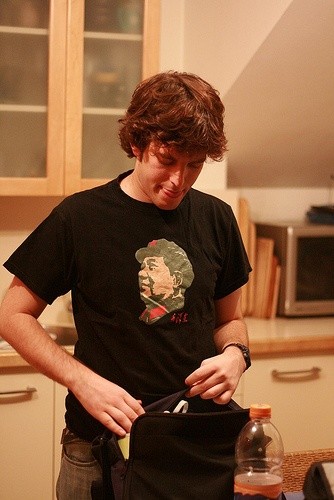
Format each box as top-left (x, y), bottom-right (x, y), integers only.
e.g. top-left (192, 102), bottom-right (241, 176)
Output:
top-left (0, 70), bottom-right (253, 500)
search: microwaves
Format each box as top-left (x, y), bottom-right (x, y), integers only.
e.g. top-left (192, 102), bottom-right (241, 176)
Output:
top-left (256, 222), bottom-right (334, 317)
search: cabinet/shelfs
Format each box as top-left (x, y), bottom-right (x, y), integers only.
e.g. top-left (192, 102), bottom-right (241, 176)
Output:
top-left (231, 337), bottom-right (334, 456)
top-left (0, 344), bottom-right (74, 500)
top-left (0, 0), bottom-right (163, 197)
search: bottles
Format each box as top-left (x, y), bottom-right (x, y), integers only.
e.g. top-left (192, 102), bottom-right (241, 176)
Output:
top-left (233, 405), bottom-right (284, 500)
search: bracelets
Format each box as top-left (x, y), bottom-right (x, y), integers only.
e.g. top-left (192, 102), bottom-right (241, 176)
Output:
top-left (222, 343), bottom-right (252, 374)
